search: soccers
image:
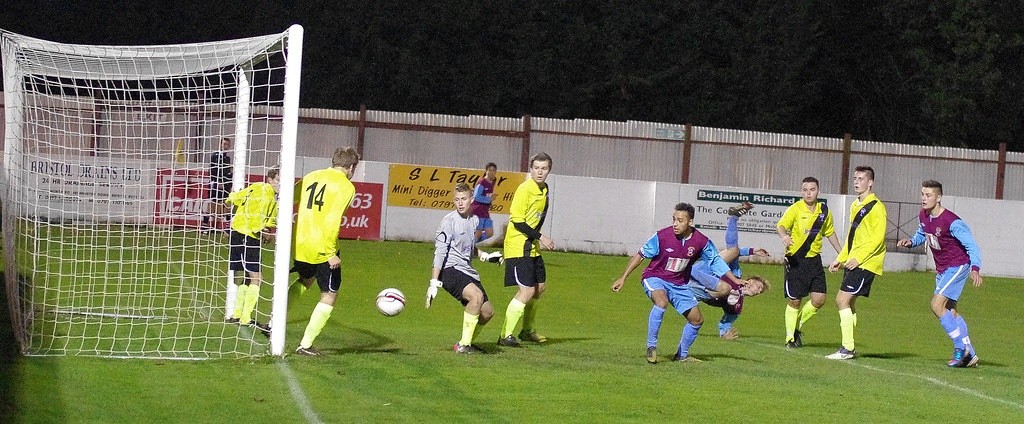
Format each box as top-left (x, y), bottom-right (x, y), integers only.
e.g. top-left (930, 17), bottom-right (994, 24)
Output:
top-left (375, 288), bottom-right (406, 317)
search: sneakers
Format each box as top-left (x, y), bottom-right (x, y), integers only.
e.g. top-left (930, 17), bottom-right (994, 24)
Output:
top-left (785, 328), bottom-right (803, 349)
top-left (518, 328), bottom-right (548, 343)
top-left (960, 354), bottom-right (979, 368)
top-left (453, 341), bottom-right (479, 354)
top-left (673, 354), bottom-right (703, 363)
top-left (498, 334), bottom-right (523, 348)
top-left (824, 346), bottom-right (857, 360)
top-left (647, 346), bottom-right (657, 363)
top-left (729, 202), bottom-right (755, 218)
top-left (948, 344), bottom-right (966, 367)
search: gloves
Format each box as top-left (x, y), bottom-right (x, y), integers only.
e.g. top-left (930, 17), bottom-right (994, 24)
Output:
top-left (480, 251), bottom-right (505, 266)
top-left (425, 279), bottom-right (443, 309)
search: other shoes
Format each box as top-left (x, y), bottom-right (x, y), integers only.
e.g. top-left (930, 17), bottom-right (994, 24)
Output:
top-left (238, 318), bottom-right (267, 329)
top-left (223, 230), bottom-right (229, 237)
top-left (201, 224), bottom-right (209, 235)
top-left (297, 345), bottom-right (321, 356)
top-left (262, 323), bottom-right (271, 337)
top-left (225, 317), bottom-right (241, 322)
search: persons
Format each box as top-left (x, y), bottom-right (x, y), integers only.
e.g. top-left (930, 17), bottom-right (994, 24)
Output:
top-left (824, 166), bottom-right (886, 360)
top-left (776, 176), bottom-right (841, 349)
top-left (498, 152), bottom-right (555, 348)
top-left (202, 138), bottom-right (233, 237)
top-left (611, 203), bottom-right (749, 363)
top-left (262, 146), bottom-right (359, 356)
top-left (225, 164), bottom-right (281, 327)
top-left (688, 202), bottom-right (770, 340)
top-left (426, 182), bottom-right (504, 356)
top-left (470, 162), bottom-right (499, 244)
top-left (897, 180), bottom-right (982, 366)
